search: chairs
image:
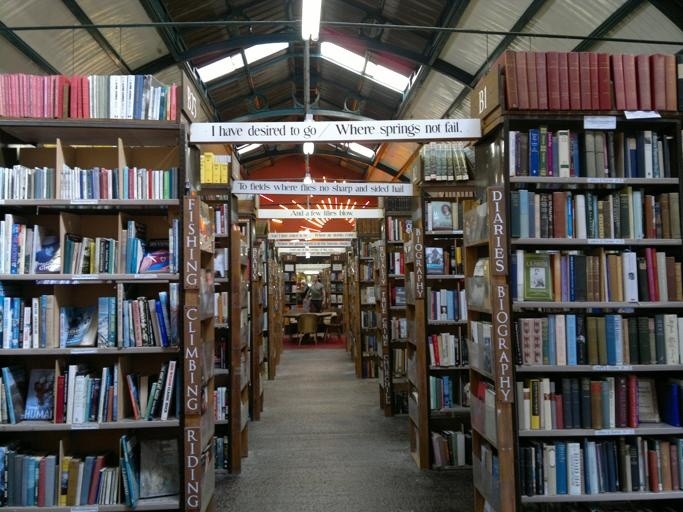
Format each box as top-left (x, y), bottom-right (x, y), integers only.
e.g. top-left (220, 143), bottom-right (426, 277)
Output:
top-left (280, 304), bottom-right (342, 348)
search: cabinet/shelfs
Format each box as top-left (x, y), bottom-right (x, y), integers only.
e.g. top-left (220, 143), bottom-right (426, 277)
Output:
top-left (185, 140), bottom-right (295, 472)
top-left (470, 62), bottom-right (683, 512)
top-left (328, 154), bottom-right (481, 470)
top-left (2, 70), bottom-right (215, 510)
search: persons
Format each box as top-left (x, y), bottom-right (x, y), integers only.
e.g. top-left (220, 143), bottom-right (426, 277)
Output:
top-left (303, 274), bottom-right (326, 313)
